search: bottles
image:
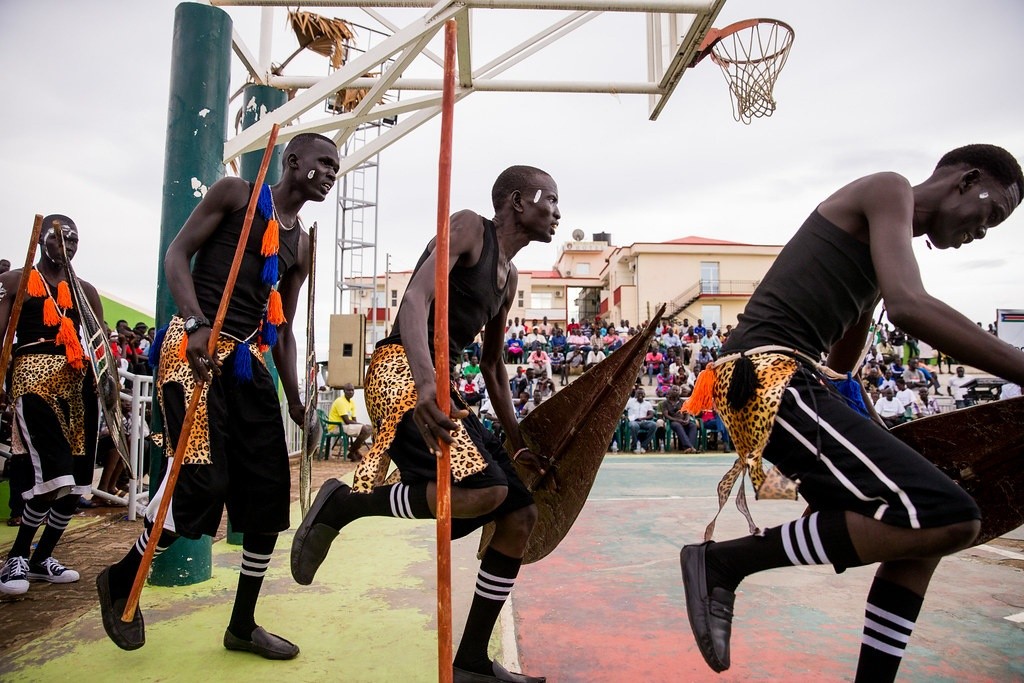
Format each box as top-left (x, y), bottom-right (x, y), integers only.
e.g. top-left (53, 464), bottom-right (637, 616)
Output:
top-left (636, 440), bottom-right (641, 455)
top-left (660, 440), bottom-right (664, 454)
top-left (613, 441), bottom-right (617, 453)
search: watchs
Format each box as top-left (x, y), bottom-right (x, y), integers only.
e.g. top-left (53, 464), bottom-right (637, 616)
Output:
top-left (183, 315), bottom-right (210, 336)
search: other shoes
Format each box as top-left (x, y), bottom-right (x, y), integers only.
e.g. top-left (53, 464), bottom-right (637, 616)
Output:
top-left (724, 444), bottom-right (731, 453)
top-left (611, 446), bottom-right (619, 452)
top-left (684, 448), bottom-right (697, 453)
top-left (290, 477), bottom-right (352, 585)
top-left (74, 508), bottom-right (83, 516)
top-left (348, 451), bottom-right (363, 461)
top-left (632, 447), bottom-right (646, 453)
top-left (7, 516), bottom-right (21, 526)
top-left (452, 659), bottom-right (546, 683)
top-left (96, 563), bottom-right (146, 651)
top-left (224, 623), bottom-right (299, 661)
top-left (717, 431), bottom-right (723, 441)
top-left (106, 488), bottom-right (130, 506)
top-left (680, 539), bottom-right (736, 674)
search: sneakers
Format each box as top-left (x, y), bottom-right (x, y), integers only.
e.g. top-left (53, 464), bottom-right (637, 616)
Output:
top-left (26, 557), bottom-right (80, 583)
top-left (0, 555), bottom-right (30, 595)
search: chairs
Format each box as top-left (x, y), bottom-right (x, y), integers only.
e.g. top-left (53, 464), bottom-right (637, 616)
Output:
top-left (318, 410), bottom-right (354, 460)
top-left (615, 400), bottom-right (723, 453)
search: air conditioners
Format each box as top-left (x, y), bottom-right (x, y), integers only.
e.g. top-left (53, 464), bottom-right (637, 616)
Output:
top-left (554, 290), bottom-right (563, 298)
top-left (358, 290), bottom-right (366, 297)
top-left (564, 269), bottom-right (573, 277)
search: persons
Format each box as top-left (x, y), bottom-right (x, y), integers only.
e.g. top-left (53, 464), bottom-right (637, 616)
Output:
top-left (97, 389), bottom-right (152, 507)
top-left (858, 318), bottom-right (954, 428)
top-left (96, 131), bottom-right (339, 662)
top-left (680, 144), bottom-right (1023, 683)
top-left (452, 329), bottom-right (506, 445)
top-left (327, 383), bottom-right (372, 461)
top-left (104, 320), bottom-right (156, 388)
top-left (977, 321), bottom-right (997, 337)
top-left (0, 215), bottom-right (110, 593)
top-left (657, 386), bottom-right (698, 453)
top-left (0, 259), bottom-right (11, 275)
top-left (640, 318), bottom-right (733, 398)
top-left (948, 366), bottom-right (977, 409)
top-left (620, 388), bottom-right (657, 454)
top-left (502, 316), bottom-right (648, 423)
top-left (699, 409), bottom-right (731, 453)
top-left (290, 164), bottom-right (560, 682)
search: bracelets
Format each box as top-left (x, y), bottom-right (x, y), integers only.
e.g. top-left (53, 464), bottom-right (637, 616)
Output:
top-left (513, 448), bottom-right (529, 460)
